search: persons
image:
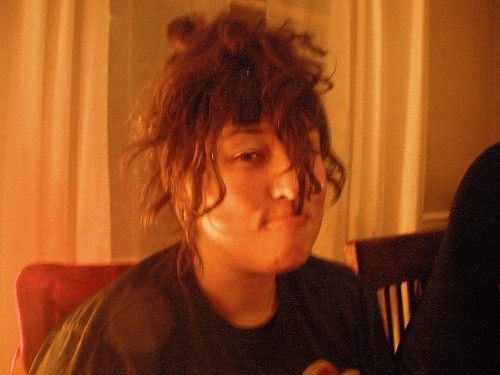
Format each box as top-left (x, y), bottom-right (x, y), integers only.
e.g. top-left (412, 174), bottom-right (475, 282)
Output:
top-left (28, 2), bottom-right (401, 375)
top-left (388, 137), bottom-right (500, 375)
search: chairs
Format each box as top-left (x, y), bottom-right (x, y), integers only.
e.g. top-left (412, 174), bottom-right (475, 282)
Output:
top-left (14, 262), bottom-right (141, 375)
top-left (352, 225), bottom-right (448, 355)
